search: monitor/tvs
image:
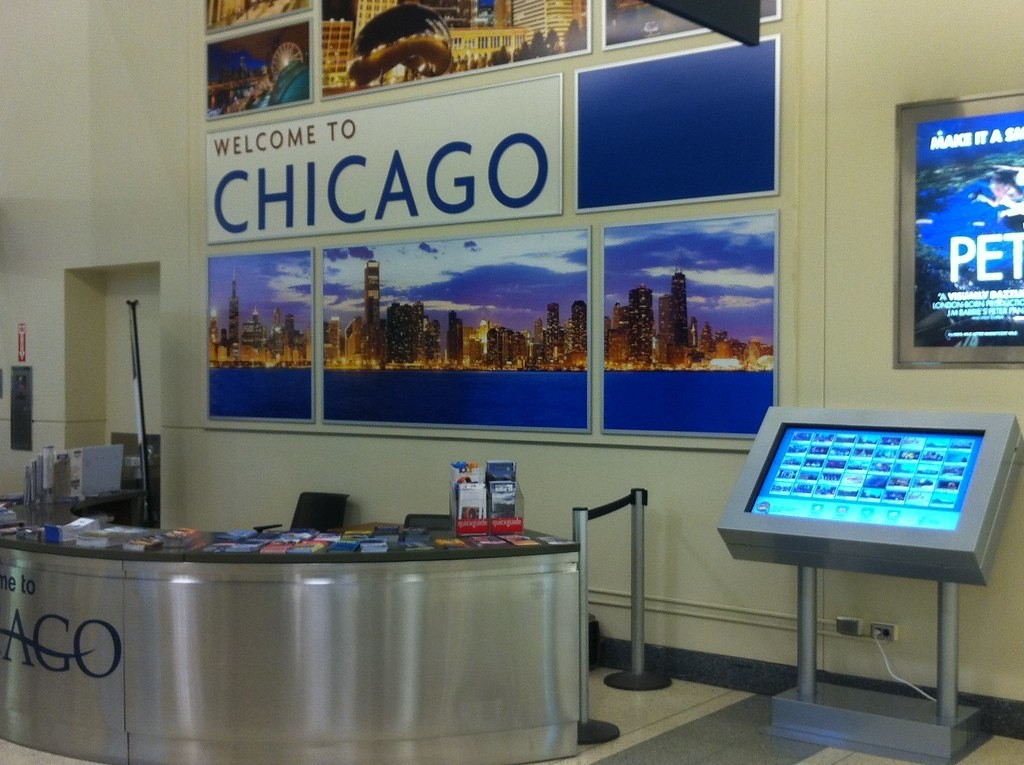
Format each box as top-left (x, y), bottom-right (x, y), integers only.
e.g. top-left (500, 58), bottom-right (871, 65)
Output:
top-left (750, 426), bottom-right (983, 530)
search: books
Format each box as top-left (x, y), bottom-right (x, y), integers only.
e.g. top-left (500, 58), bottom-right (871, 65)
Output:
top-left (123, 527), bottom-right (579, 554)
top-left (77, 525), bottom-right (149, 548)
top-left (451, 461), bottom-right (516, 536)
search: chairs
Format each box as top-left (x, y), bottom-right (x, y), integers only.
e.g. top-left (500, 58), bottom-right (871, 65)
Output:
top-left (254, 492), bottom-right (349, 534)
top-left (404, 514), bottom-right (452, 530)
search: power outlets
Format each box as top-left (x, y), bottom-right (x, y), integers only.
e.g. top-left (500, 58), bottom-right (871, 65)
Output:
top-left (870, 623), bottom-right (898, 642)
